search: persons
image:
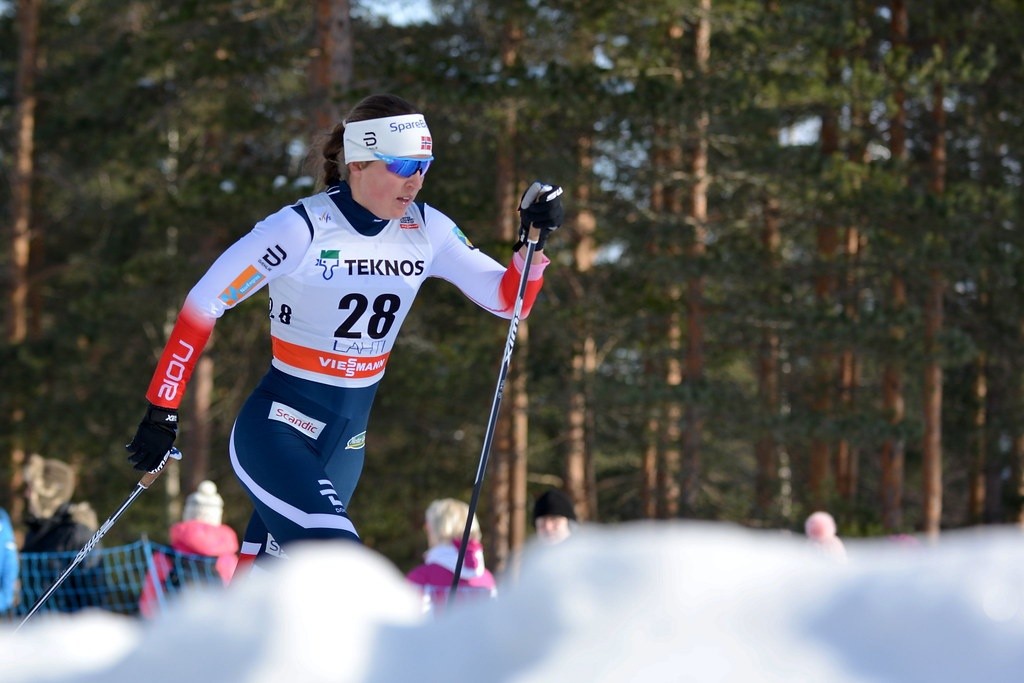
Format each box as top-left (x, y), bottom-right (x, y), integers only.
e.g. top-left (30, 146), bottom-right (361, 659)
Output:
top-left (137, 480), bottom-right (239, 621)
top-left (10, 459), bottom-right (103, 613)
top-left (531, 486), bottom-right (578, 546)
top-left (123, 95), bottom-right (566, 590)
top-left (0, 507), bottom-right (16, 616)
top-left (405, 499), bottom-right (497, 612)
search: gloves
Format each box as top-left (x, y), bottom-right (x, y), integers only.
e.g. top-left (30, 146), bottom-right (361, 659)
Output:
top-left (126, 403), bottom-right (183, 474)
top-left (511, 181), bottom-right (564, 252)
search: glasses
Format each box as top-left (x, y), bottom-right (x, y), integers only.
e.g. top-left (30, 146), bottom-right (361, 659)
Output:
top-left (346, 138), bottom-right (435, 178)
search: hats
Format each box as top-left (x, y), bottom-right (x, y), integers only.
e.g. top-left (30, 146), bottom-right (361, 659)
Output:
top-left (24, 456), bottom-right (75, 526)
top-left (532, 491), bottom-right (577, 527)
top-left (182, 481), bottom-right (224, 527)
